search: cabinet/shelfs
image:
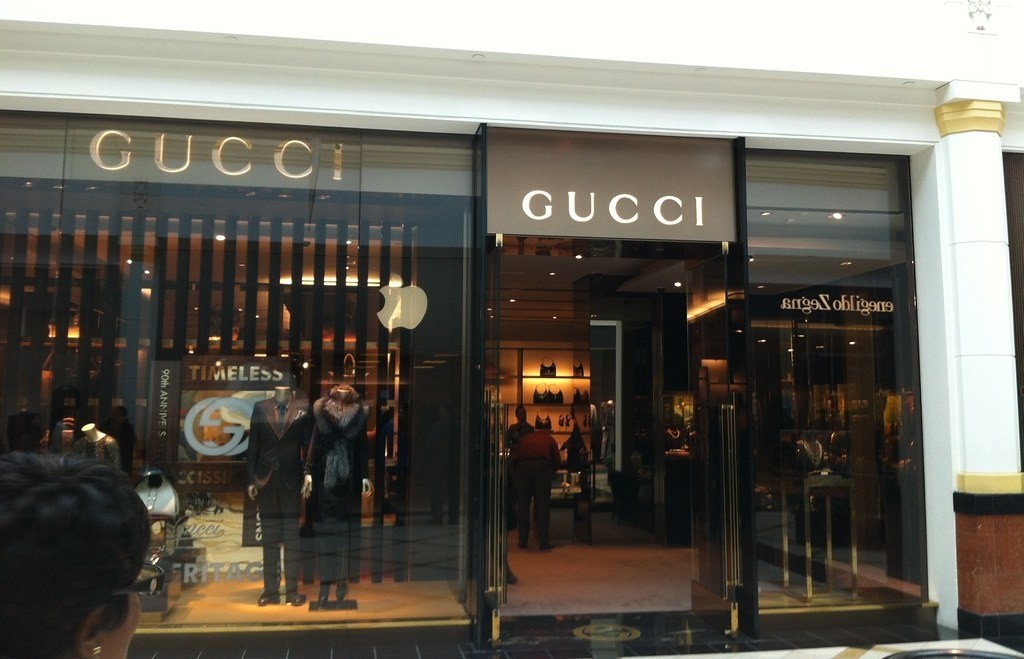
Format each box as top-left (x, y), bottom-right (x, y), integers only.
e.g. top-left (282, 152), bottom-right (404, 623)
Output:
top-left (481, 347), bottom-right (750, 512)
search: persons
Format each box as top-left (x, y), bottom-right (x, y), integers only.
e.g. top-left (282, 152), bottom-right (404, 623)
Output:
top-left (6, 397), bottom-right (42, 450)
top-left (301, 384), bottom-right (374, 606)
top-left (507, 406), bottom-right (534, 451)
top-left (73, 423), bottom-right (121, 467)
top-left (512, 433), bottom-right (561, 551)
top-left (99, 406), bottom-right (138, 472)
top-left (246, 386), bottom-right (312, 606)
top-left (0, 450), bottom-right (151, 659)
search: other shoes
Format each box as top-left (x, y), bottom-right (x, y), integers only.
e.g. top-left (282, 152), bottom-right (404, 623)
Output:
top-left (540, 544), bottom-right (554, 550)
top-left (507, 569), bottom-right (517, 583)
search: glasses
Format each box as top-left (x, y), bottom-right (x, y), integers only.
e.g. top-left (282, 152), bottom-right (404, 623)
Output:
top-left (92, 563), bottom-right (164, 598)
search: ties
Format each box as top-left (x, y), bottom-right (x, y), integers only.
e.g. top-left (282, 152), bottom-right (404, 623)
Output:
top-left (278, 404), bottom-right (285, 433)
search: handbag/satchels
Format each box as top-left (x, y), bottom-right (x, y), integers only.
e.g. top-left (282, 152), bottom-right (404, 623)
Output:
top-left (534, 383), bottom-right (589, 403)
top-left (540, 355), bottom-right (556, 376)
top-left (360, 477), bottom-right (375, 516)
top-left (534, 408), bottom-right (551, 429)
top-left (573, 362), bottom-right (583, 376)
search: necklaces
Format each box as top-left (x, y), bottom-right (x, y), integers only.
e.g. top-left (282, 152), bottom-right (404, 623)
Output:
top-left (802, 438), bottom-right (822, 467)
top-left (669, 429), bottom-right (680, 438)
